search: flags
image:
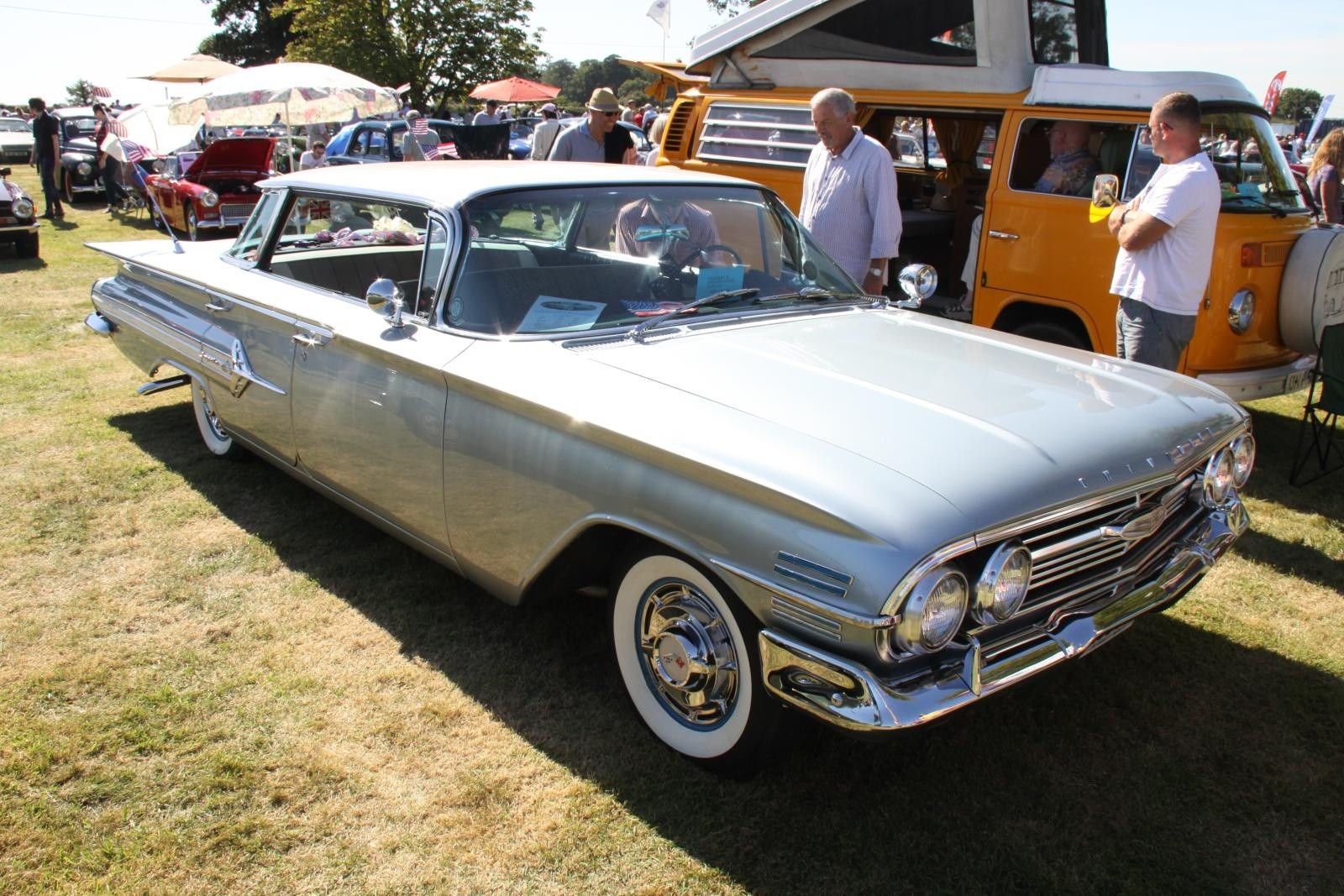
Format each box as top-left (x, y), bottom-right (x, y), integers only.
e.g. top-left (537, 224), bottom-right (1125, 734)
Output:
top-left (106, 114), bottom-right (128, 138)
top-left (91, 86), bottom-right (111, 98)
top-left (122, 140), bottom-right (166, 164)
top-left (620, 299), bottom-right (698, 316)
top-left (392, 83), bottom-right (461, 161)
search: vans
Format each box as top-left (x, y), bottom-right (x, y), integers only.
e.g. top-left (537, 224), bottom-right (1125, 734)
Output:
top-left (615, 0), bottom-right (1344, 399)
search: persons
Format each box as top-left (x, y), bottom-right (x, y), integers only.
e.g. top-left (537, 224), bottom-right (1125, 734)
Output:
top-left (899, 121), bottom-right (912, 134)
top-left (442, 88), bottom-right (723, 270)
top-left (109, 99), bottom-right (133, 111)
top-left (1200, 132), bottom-right (1306, 162)
top-left (1305, 127), bottom-right (1344, 223)
top-left (273, 113), bottom-right (280, 124)
top-left (1, 107), bottom-right (27, 119)
top-left (938, 120), bottom-right (1103, 321)
top-left (798, 88), bottom-right (903, 295)
top-left (89, 103), bottom-right (136, 214)
top-left (28, 98), bottom-right (65, 219)
top-left (404, 101), bottom-right (410, 115)
top-left (299, 123), bottom-right (332, 170)
top-left (1107, 92), bottom-right (1222, 370)
top-left (403, 110), bottom-right (444, 162)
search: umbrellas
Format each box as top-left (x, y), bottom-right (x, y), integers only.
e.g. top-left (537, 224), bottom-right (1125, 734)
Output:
top-left (468, 76), bottom-right (562, 118)
top-left (136, 53), bottom-right (404, 173)
top-left (100, 99), bottom-right (205, 163)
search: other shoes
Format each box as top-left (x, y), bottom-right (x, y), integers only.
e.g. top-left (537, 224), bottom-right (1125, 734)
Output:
top-left (53, 212), bottom-right (64, 217)
top-left (36, 213), bottom-right (55, 219)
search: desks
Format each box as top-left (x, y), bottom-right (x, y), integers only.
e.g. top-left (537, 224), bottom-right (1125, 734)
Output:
top-left (900, 208), bottom-right (953, 240)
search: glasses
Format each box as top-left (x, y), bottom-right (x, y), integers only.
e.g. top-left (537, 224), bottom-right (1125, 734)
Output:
top-left (1044, 128), bottom-right (1063, 138)
top-left (1145, 123), bottom-right (1173, 134)
top-left (601, 112), bottom-right (621, 118)
top-left (654, 200), bottom-right (684, 206)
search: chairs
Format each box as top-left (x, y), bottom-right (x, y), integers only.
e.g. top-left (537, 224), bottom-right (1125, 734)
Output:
top-left (116, 180), bottom-right (151, 220)
top-left (1287, 322), bottom-right (1344, 488)
top-left (1076, 130), bottom-right (1134, 198)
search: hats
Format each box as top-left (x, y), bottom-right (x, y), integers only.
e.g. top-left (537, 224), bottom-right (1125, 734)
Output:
top-left (649, 165), bottom-right (687, 201)
top-left (404, 109), bottom-right (422, 120)
top-left (539, 103), bottom-right (558, 115)
top-left (1299, 133), bottom-right (1305, 138)
top-left (586, 86), bottom-right (626, 113)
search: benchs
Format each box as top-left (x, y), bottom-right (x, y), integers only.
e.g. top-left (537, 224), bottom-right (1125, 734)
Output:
top-left (458, 264), bottom-right (652, 326)
top-left (269, 243), bottom-right (539, 307)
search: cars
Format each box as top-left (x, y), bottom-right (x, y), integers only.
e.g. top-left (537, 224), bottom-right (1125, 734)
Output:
top-left (0, 106), bottom-right (653, 259)
top-left (1203, 141), bottom-right (1310, 184)
top-left (894, 129), bottom-right (997, 168)
top-left (85, 160), bottom-right (1255, 772)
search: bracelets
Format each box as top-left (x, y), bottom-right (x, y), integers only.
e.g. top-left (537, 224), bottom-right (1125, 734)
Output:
top-left (1121, 209), bottom-right (1130, 223)
top-left (870, 268), bottom-right (884, 276)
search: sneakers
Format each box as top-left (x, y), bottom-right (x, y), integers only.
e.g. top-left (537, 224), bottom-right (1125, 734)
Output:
top-left (102, 203), bottom-right (118, 215)
top-left (939, 301), bottom-right (973, 321)
top-left (123, 200), bottom-right (134, 212)
